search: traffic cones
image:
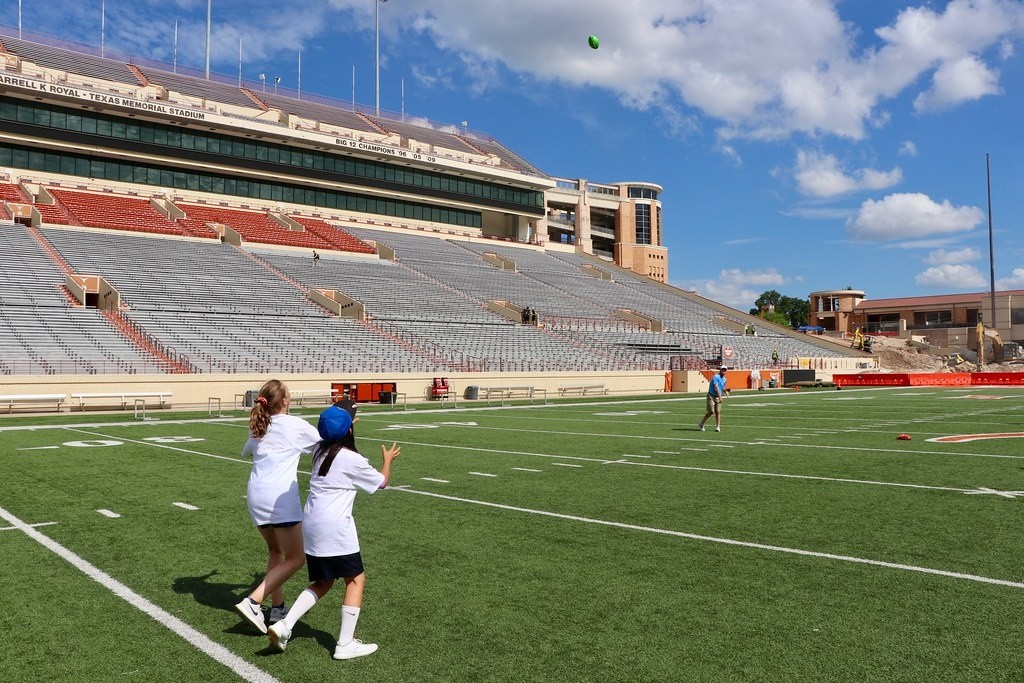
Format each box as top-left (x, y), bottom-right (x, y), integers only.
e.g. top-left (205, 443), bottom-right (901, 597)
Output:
top-left (795, 385), bottom-right (798, 392)
top-left (837, 380), bottom-right (842, 390)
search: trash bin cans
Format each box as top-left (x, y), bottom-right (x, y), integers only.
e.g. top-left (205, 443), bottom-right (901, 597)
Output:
top-left (467, 386), bottom-right (479, 400)
top-left (379, 392), bottom-right (397, 404)
top-left (246, 390), bottom-right (259, 407)
top-left (769, 381), bottom-right (777, 388)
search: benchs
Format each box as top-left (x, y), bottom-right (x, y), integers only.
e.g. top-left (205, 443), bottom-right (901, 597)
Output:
top-left (0, 184), bottom-right (861, 414)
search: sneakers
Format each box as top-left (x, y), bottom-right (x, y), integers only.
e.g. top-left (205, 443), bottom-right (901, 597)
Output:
top-left (235, 597), bottom-right (268, 634)
top-left (333, 637), bottom-right (378, 659)
top-left (270, 605), bottom-right (290, 625)
top-left (268, 618), bottom-right (292, 652)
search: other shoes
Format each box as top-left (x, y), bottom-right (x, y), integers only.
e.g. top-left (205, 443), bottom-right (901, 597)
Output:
top-left (698, 421), bottom-right (705, 432)
top-left (714, 426), bottom-right (720, 432)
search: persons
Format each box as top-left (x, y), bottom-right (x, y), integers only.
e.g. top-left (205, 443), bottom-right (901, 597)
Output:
top-left (234, 379), bottom-right (359, 634)
top-left (520, 306), bottom-right (536, 326)
top-left (744, 322), bottom-right (748, 334)
top-left (824, 328), bottom-right (826, 332)
top-left (868, 337), bottom-right (871, 341)
top-left (772, 348), bottom-right (779, 366)
top-left (313, 251), bottom-right (320, 265)
top-left (267, 400), bottom-right (401, 660)
top-left (751, 324), bottom-right (755, 334)
top-left (698, 365), bottom-right (729, 432)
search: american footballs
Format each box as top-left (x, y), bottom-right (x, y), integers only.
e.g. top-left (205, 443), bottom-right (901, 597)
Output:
top-left (589, 36), bottom-right (599, 49)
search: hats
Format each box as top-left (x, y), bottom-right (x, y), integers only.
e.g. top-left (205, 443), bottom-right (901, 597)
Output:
top-left (720, 365), bottom-right (728, 370)
top-left (532, 307), bottom-right (534, 310)
top-left (317, 399), bottom-right (357, 443)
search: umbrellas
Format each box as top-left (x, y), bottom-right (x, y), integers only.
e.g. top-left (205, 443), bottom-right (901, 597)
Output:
top-left (799, 326), bottom-right (816, 335)
top-left (812, 326), bottom-right (824, 335)
